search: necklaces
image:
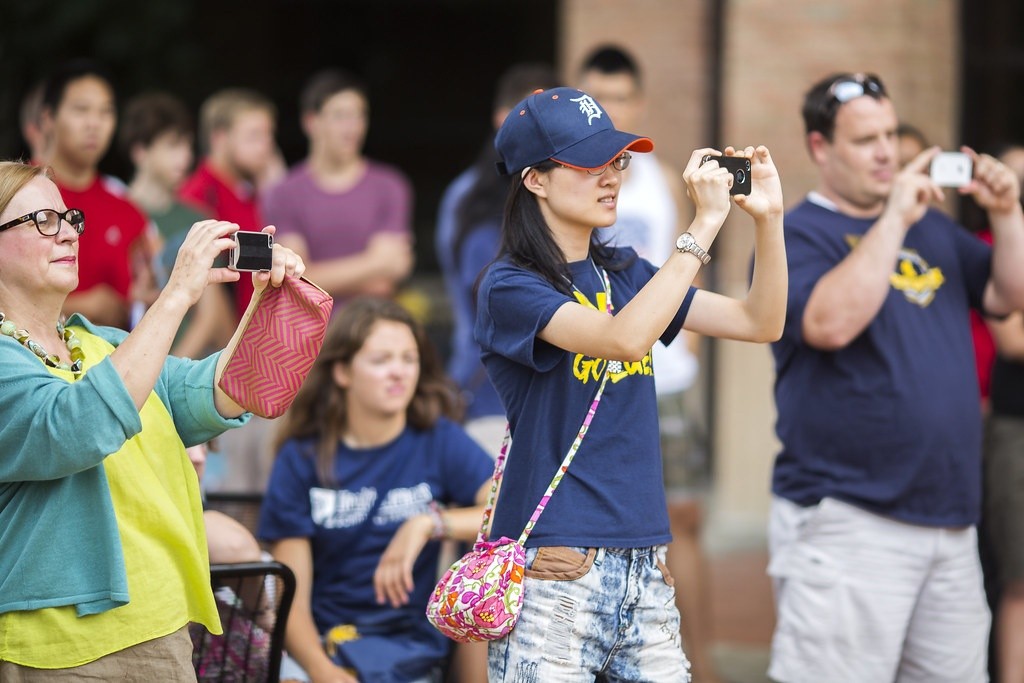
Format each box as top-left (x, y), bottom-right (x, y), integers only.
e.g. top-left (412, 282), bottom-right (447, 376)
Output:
top-left (0, 311), bottom-right (86, 381)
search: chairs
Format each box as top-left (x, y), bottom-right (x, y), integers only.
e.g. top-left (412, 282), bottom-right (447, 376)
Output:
top-left (187, 560), bottom-right (296, 683)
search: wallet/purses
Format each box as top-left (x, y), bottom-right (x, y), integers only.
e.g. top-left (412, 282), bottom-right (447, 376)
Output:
top-left (217, 275), bottom-right (333, 419)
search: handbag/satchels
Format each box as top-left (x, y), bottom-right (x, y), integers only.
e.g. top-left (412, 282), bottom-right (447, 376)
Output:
top-left (426, 537), bottom-right (525, 645)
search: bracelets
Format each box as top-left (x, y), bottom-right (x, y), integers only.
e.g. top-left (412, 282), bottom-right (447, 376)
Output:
top-left (247, 601), bottom-right (273, 618)
top-left (418, 500), bottom-right (449, 540)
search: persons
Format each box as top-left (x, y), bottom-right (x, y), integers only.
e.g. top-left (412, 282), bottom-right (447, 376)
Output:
top-left (0, 160), bottom-right (308, 683)
top-left (182, 424), bottom-right (284, 683)
top-left (253, 294), bottom-right (503, 683)
top-left (746, 68), bottom-right (1024, 683)
top-left (0, 58), bottom-right (415, 493)
top-left (433, 38), bottom-right (790, 683)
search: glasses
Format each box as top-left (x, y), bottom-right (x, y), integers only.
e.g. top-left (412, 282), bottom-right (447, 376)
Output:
top-left (544, 152), bottom-right (632, 176)
top-left (814, 75), bottom-right (882, 128)
top-left (0, 208), bottom-right (85, 236)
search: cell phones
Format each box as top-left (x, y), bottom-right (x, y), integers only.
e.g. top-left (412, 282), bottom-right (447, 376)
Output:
top-left (230, 231), bottom-right (274, 271)
top-left (929, 151), bottom-right (973, 188)
top-left (702, 155), bottom-right (751, 196)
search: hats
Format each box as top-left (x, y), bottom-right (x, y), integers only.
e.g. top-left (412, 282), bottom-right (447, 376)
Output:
top-left (493, 87), bottom-right (655, 178)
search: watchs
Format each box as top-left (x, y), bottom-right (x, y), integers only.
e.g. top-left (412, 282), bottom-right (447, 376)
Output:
top-left (675, 231), bottom-right (712, 265)
